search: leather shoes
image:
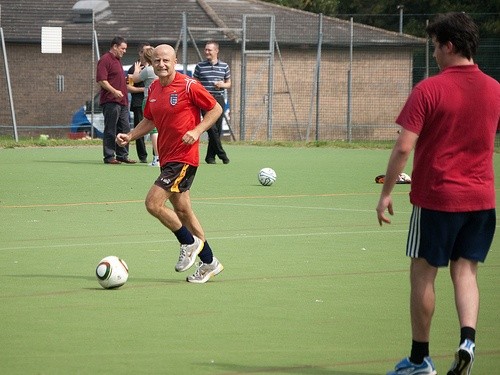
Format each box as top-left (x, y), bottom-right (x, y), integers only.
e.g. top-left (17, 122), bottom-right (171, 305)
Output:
top-left (116, 156), bottom-right (137, 164)
top-left (104, 159), bottom-right (121, 165)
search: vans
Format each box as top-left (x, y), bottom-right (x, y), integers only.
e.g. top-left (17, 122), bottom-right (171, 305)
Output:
top-left (65, 64), bottom-right (230, 140)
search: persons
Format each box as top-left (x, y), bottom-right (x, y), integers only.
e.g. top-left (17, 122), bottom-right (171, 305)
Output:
top-left (193, 42), bottom-right (231, 164)
top-left (375, 13), bottom-right (500, 375)
top-left (96, 37), bottom-right (159, 166)
top-left (117, 45), bottom-right (224, 283)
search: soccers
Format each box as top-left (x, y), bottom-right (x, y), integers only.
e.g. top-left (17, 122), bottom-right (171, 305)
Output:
top-left (258, 168), bottom-right (277, 186)
top-left (96, 256), bottom-right (129, 289)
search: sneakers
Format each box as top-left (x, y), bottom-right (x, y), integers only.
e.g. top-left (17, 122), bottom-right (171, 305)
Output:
top-left (447, 338), bottom-right (477, 375)
top-left (186, 256), bottom-right (224, 283)
top-left (174, 234), bottom-right (204, 273)
top-left (386, 355), bottom-right (438, 375)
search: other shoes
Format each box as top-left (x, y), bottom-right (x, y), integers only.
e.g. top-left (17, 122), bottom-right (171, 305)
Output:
top-left (219, 154), bottom-right (230, 164)
top-left (205, 156), bottom-right (217, 164)
